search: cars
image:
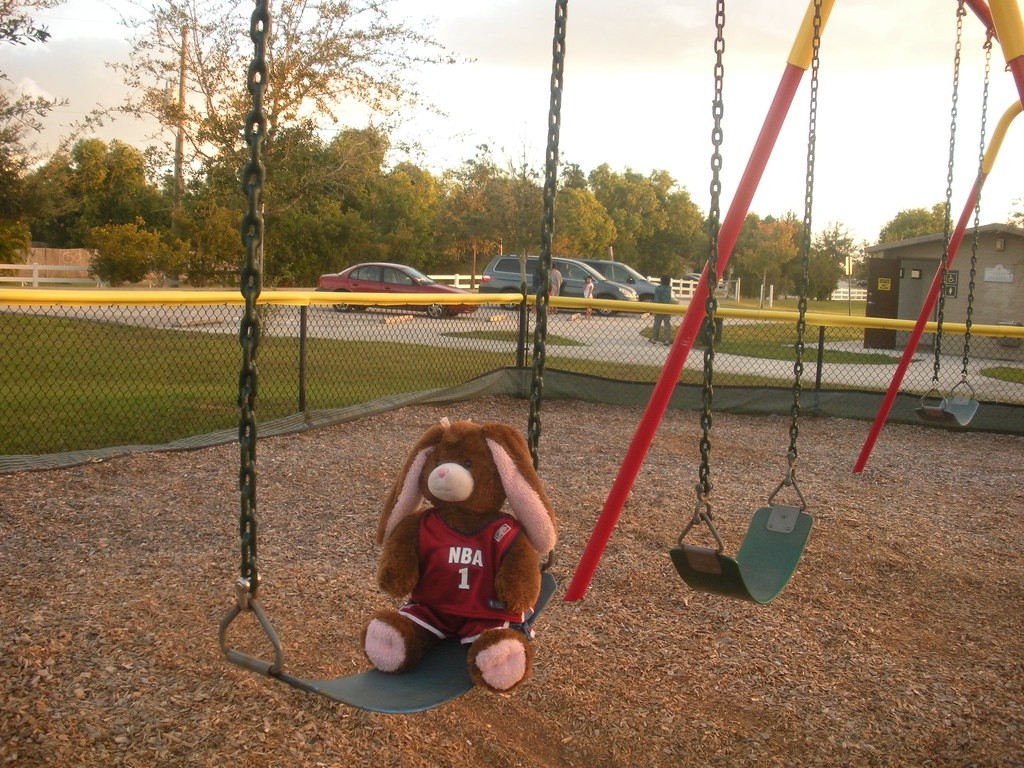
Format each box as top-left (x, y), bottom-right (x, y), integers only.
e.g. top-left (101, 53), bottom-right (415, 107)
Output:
top-left (479, 255), bottom-right (680, 316)
top-left (318, 263), bottom-right (481, 319)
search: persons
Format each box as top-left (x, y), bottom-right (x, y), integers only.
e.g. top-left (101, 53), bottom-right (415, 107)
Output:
top-left (547, 262), bottom-right (563, 315)
top-left (584, 276), bottom-right (594, 320)
top-left (648, 274), bottom-right (672, 346)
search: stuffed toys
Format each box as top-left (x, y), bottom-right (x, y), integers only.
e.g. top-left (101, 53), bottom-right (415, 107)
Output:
top-left (361, 417), bottom-right (558, 693)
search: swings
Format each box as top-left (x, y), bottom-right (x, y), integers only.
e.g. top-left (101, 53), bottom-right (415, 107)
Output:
top-left (915, 1), bottom-right (996, 433)
top-left (668, 1), bottom-right (825, 609)
top-left (219, 0), bottom-right (571, 719)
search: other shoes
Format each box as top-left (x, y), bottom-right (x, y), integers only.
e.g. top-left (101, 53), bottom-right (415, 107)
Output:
top-left (648, 339), bottom-right (656, 344)
top-left (582, 316), bottom-right (592, 320)
top-left (663, 342), bottom-right (669, 346)
